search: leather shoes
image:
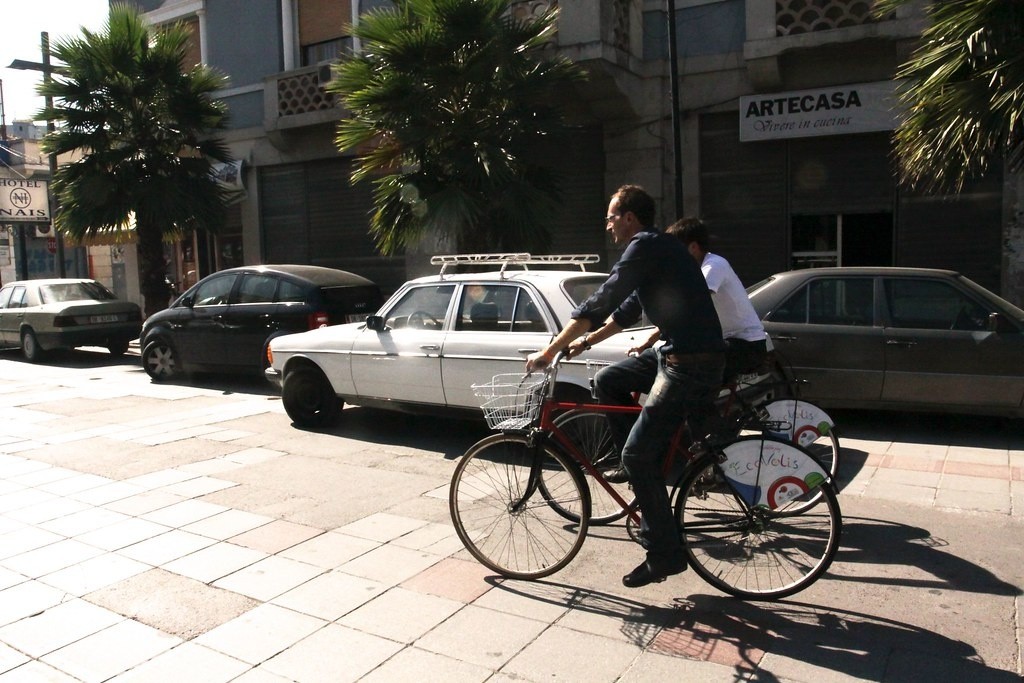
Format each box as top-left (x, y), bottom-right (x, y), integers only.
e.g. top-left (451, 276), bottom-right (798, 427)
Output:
top-left (603, 466), bottom-right (629, 483)
top-left (623, 558), bottom-right (688, 587)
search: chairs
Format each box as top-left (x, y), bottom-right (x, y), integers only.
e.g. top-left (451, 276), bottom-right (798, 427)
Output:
top-left (470, 302), bottom-right (498, 329)
top-left (241, 283), bottom-right (274, 303)
top-left (525, 301), bottom-right (545, 331)
top-left (851, 297), bottom-right (873, 326)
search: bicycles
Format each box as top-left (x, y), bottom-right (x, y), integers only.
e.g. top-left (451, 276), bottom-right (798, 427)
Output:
top-left (537, 349), bottom-right (840, 527)
top-left (448, 333), bottom-right (842, 601)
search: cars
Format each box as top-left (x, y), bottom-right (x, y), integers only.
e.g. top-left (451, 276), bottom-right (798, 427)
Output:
top-left (0, 278), bottom-right (143, 363)
top-left (265, 252), bottom-right (669, 427)
top-left (744, 266), bottom-right (1024, 436)
top-left (139, 264), bottom-right (385, 382)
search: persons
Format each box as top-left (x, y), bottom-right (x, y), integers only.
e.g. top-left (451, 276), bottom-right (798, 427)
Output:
top-left (524, 185), bottom-right (723, 588)
top-left (627, 214), bottom-right (769, 379)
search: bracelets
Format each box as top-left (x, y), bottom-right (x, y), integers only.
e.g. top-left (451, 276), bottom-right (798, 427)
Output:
top-left (580, 338), bottom-right (592, 351)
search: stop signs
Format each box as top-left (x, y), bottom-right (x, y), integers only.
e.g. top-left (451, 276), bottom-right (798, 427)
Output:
top-left (46, 238), bottom-right (56, 254)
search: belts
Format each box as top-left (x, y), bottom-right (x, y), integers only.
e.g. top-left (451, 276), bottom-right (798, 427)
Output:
top-left (668, 352), bottom-right (725, 363)
